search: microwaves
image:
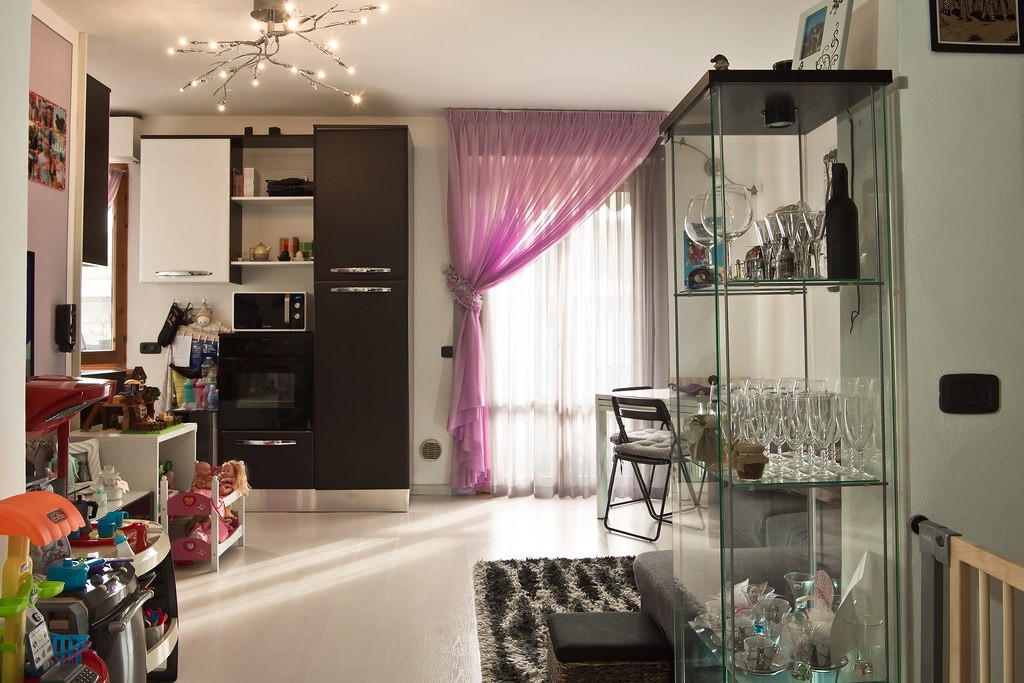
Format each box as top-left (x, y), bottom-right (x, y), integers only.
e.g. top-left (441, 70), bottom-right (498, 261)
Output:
top-left (232, 292), bottom-right (306, 332)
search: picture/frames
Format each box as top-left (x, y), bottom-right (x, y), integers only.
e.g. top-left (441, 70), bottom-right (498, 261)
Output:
top-left (793, 0), bottom-right (853, 70)
top-left (929, 0), bottom-right (1024, 54)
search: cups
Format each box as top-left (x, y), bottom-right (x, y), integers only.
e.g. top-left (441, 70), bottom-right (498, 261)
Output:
top-left (706, 572), bottom-right (814, 682)
top-left (145, 623), bottom-right (164, 650)
top-left (842, 614), bottom-right (884, 677)
top-left (68, 528), bottom-right (80, 539)
top-left (107, 511), bottom-right (129, 527)
top-left (98, 518), bottom-right (117, 538)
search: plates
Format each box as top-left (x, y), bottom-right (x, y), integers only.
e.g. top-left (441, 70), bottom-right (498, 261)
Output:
top-left (117, 520), bottom-right (133, 528)
top-left (694, 615), bottom-right (792, 676)
top-left (89, 530), bottom-right (124, 540)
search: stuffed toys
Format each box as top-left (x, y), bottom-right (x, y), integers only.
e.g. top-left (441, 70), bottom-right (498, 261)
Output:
top-left (189, 303), bottom-right (231, 335)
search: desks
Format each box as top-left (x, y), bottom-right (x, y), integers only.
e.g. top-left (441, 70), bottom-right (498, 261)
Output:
top-left (597, 388), bottom-right (698, 520)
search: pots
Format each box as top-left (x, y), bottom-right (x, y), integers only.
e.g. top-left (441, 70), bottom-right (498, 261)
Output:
top-left (46, 558), bottom-right (105, 592)
top-left (72, 557), bottom-right (134, 570)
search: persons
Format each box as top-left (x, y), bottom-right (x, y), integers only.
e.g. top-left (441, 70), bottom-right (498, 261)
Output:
top-left (192, 462), bottom-right (212, 492)
top-left (710, 54), bottom-right (730, 70)
top-left (219, 460), bottom-right (252, 497)
top-left (29, 91), bottom-right (65, 189)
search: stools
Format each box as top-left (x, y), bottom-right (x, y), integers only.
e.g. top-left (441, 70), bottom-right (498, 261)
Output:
top-left (547, 610), bottom-right (673, 683)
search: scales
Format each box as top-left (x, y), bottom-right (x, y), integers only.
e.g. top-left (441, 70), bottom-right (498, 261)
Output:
top-left (40, 661), bottom-right (100, 683)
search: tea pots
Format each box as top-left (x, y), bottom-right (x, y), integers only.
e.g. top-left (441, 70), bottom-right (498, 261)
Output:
top-left (249, 242), bottom-right (272, 261)
top-left (71, 495), bottom-right (98, 533)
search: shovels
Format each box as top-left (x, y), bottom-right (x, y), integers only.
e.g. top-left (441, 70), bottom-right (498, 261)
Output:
top-left (74, 556), bottom-right (134, 571)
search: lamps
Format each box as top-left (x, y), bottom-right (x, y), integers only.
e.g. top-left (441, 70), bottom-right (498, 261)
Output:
top-left (661, 136), bottom-right (759, 199)
top-left (167, 0), bottom-right (389, 112)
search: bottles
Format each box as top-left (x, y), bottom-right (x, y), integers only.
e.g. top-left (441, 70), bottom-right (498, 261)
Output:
top-left (184, 357), bottom-right (219, 409)
top-left (776, 237), bottom-right (795, 280)
top-left (826, 163), bottom-right (860, 279)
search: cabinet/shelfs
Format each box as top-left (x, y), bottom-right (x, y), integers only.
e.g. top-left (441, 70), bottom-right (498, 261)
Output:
top-left (229, 133), bottom-right (313, 266)
top-left (313, 122), bottom-right (415, 491)
top-left (659, 67), bottom-right (895, 683)
top-left (71, 518), bottom-right (179, 683)
top-left (69, 423), bottom-right (197, 523)
top-left (222, 430), bottom-right (314, 490)
top-left (137, 135), bottom-right (242, 286)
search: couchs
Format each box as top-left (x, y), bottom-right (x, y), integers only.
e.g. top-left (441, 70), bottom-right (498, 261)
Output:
top-left (633, 485), bottom-right (842, 667)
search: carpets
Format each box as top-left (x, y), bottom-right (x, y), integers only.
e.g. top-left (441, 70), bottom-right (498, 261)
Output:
top-left (471, 555), bottom-right (641, 683)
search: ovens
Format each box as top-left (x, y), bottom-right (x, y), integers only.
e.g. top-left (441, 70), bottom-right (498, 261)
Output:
top-left (218, 332), bottom-right (314, 431)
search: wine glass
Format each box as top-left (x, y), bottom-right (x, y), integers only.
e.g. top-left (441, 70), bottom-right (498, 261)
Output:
top-left (697, 377), bottom-right (874, 480)
top-left (684, 184), bottom-right (752, 291)
top-left (753, 209), bottom-right (826, 280)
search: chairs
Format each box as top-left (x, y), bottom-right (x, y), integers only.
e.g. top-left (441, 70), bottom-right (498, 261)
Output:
top-left (609, 386), bottom-right (697, 516)
top-left (605, 395), bottom-right (706, 543)
